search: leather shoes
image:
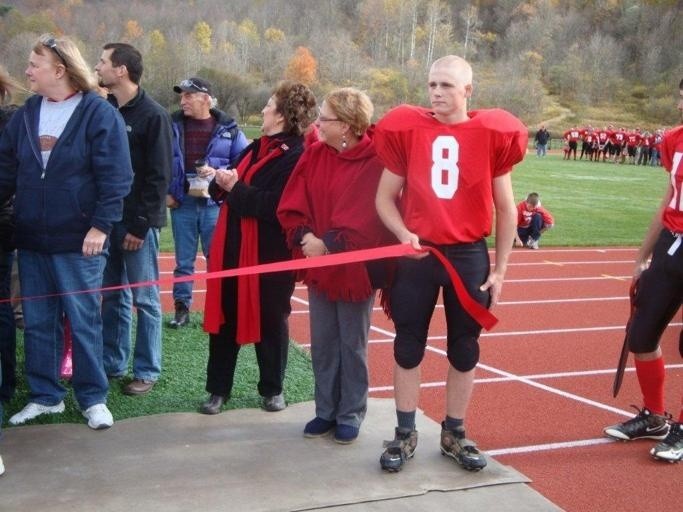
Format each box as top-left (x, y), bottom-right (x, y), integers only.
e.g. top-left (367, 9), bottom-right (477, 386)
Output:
top-left (201, 394), bottom-right (222, 414)
top-left (261, 393), bottom-right (284, 410)
top-left (123, 379), bottom-right (153, 394)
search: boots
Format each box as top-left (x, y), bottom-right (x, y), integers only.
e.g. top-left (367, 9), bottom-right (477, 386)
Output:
top-left (169, 301), bottom-right (189, 328)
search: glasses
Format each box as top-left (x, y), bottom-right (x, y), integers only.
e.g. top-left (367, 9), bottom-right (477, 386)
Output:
top-left (317, 106), bottom-right (342, 121)
top-left (40, 33), bottom-right (67, 65)
top-left (180, 79), bottom-right (204, 91)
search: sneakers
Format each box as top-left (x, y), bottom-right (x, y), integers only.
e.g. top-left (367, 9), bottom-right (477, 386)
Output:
top-left (380, 424), bottom-right (417, 472)
top-left (304, 416), bottom-right (333, 437)
top-left (527, 235), bottom-right (538, 249)
top-left (335, 424), bottom-right (358, 444)
top-left (650, 423), bottom-right (683, 463)
top-left (8, 400), bottom-right (65, 425)
top-left (82, 403), bottom-right (113, 429)
top-left (603, 407), bottom-right (670, 440)
top-left (440, 421), bottom-right (486, 471)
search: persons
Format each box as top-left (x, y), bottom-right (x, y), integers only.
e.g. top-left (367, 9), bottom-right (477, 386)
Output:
top-left (534, 125), bottom-right (551, 158)
top-left (1, 30), bottom-right (133, 430)
top-left (374, 54), bottom-right (529, 472)
top-left (562, 124), bottom-right (670, 167)
top-left (166, 78), bottom-right (249, 329)
top-left (275, 86), bottom-right (402, 443)
top-left (95, 42), bottom-right (174, 397)
top-left (516, 192), bottom-right (554, 250)
top-left (602, 80), bottom-right (683, 462)
top-left (200, 83), bottom-right (318, 416)
top-left (1, 68), bottom-right (21, 408)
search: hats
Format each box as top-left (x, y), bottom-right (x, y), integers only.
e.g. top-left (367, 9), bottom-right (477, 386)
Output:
top-left (174, 78), bottom-right (210, 94)
